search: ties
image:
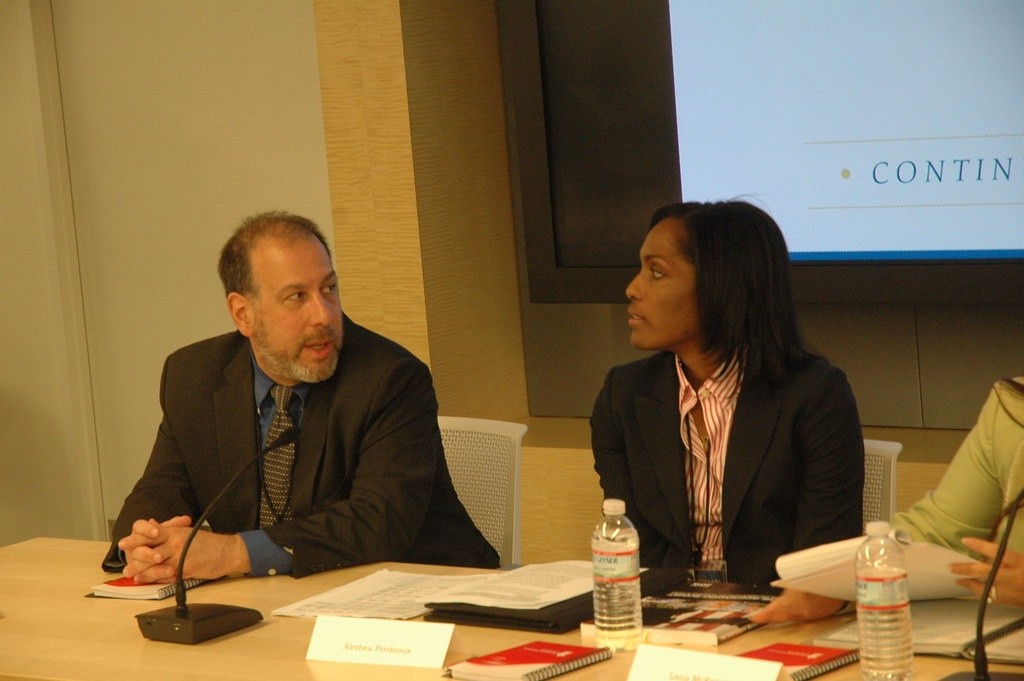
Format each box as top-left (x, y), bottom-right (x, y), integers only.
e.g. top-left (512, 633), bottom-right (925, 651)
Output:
top-left (257, 384), bottom-right (296, 530)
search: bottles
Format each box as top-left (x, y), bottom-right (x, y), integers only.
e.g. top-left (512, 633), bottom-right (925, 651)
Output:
top-left (590, 498), bottom-right (643, 652)
top-left (855, 521), bottom-right (914, 681)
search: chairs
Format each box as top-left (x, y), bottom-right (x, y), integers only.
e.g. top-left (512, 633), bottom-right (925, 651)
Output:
top-left (862, 439), bottom-right (902, 526)
top-left (436, 416), bottom-right (528, 571)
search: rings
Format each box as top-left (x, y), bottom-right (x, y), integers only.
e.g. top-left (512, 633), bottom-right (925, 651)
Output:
top-left (986, 584), bottom-right (996, 605)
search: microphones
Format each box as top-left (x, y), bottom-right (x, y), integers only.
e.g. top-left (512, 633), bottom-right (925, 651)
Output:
top-left (135, 425), bottom-right (303, 645)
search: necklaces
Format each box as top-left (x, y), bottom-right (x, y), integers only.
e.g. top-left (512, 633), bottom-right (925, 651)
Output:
top-left (691, 411), bottom-right (710, 455)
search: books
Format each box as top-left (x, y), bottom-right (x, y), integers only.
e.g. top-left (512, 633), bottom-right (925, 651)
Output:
top-left (772, 535), bottom-right (981, 604)
top-left (736, 643), bottom-right (860, 681)
top-left (446, 640), bottom-right (612, 681)
top-left (93, 577), bottom-right (208, 600)
top-left (581, 593), bottom-right (778, 645)
top-left (423, 558), bottom-right (666, 634)
top-left (812, 597), bottom-right (1024, 667)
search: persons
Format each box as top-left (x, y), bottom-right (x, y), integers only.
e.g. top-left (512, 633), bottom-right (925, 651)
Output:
top-left (743, 373), bottom-right (1024, 625)
top-left (588, 202), bottom-right (867, 594)
top-left (102, 213), bottom-right (502, 581)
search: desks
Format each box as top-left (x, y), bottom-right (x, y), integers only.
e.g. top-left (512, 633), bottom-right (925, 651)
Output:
top-left (0, 535), bottom-right (1023, 681)
